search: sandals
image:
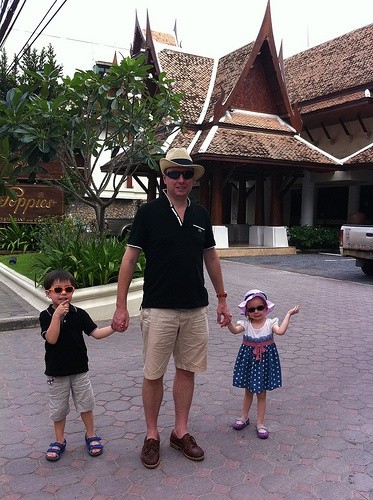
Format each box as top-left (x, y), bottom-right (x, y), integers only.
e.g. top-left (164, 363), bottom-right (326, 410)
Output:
top-left (46, 438), bottom-right (66, 461)
top-left (85, 432), bottom-right (104, 457)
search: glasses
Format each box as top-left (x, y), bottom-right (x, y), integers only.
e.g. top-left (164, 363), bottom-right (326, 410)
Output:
top-left (246, 305), bottom-right (266, 313)
top-left (165, 170), bottom-right (194, 180)
top-left (48, 286), bottom-right (74, 293)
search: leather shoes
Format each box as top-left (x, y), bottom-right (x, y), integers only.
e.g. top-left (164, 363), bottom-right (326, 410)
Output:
top-left (140, 435), bottom-right (160, 469)
top-left (169, 429), bottom-right (204, 461)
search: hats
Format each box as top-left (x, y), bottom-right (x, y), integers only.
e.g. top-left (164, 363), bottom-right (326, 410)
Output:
top-left (238, 289), bottom-right (275, 312)
top-left (159, 147), bottom-right (205, 181)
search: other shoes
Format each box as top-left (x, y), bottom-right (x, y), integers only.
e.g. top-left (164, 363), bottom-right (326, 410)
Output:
top-left (256, 426), bottom-right (268, 439)
top-left (232, 418), bottom-right (249, 430)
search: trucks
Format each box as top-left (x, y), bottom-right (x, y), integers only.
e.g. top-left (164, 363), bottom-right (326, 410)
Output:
top-left (339, 224), bottom-right (373, 276)
top-left (103, 216), bottom-right (134, 240)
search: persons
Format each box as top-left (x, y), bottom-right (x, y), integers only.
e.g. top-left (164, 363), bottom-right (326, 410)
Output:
top-left (223, 289), bottom-right (300, 438)
top-left (38, 269), bottom-right (125, 461)
top-left (110, 148), bottom-right (232, 469)
